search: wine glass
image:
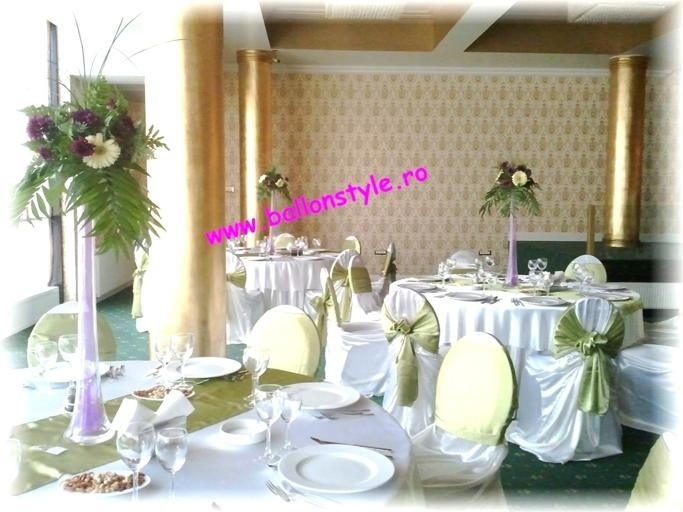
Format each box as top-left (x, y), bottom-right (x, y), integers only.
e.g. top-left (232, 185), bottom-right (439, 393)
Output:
top-left (156, 428), bottom-right (186, 493)
top-left (149, 333), bottom-right (194, 384)
top-left (242, 346), bottom-right (270, 408)
top-left (226, 231), bottom-right (321, 255)
top-left (253, 384), bottom-right (281, 467)
top-left (31, 333), bottom-right (79, 392)
top-left (280, 387), bottom-right (303, 452)
top-left (435, 255), bottom-right (590, 306)
top-left (116, 422), bottom-right (155, 498)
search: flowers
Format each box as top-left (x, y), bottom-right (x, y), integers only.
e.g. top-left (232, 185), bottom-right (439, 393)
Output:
top-left (6, 12), bottom-right (173, 261)
top-left (252, 162), bottom-right (297, 206)
top-left (477, 159), bottom-right (545, 221)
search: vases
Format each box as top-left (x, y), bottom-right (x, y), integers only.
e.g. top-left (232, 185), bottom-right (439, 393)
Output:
top-left (264, 189), bottom-right (277, 254)
top-left (503, 193), bottom-right (521, 287)
top-left (63, 205), bottom-right (119, 448)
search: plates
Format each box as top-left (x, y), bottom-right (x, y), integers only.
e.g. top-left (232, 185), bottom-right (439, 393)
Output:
top-left (239, 255), bottom-right (325, 262)
top-left (277, 443), bottom-right (395, 496)
top-left (406, 273), bottom-right (447, 283)
top-left (396, 283), bottom-right (435, 293)
top-left (280, 382), bottom-right (360, 409)
top-left (57, 470), bottom-right (150, 495)
top-left (582, 293), bottom-right (631, 302)
top-left (593, 283), bottom-right (626, 291)
top-left (132, 390), bottom-right (195, 401)
top-left (26, 360), bottom-right (112, 382)
top-left (175, 356), bottom-right (240, 378)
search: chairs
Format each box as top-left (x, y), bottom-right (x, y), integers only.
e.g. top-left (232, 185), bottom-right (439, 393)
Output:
top-left (5, 230), bottom-right (678, 508)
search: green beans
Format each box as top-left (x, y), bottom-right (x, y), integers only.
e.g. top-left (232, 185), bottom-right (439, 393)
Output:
top-left (64, 472), bottom-right (145, 493)
top-left (134, 386), bottom-right (189, 398)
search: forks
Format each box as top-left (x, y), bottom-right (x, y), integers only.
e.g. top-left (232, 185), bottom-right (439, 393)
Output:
top-left (264, 478), bottom-right (286, 496)
